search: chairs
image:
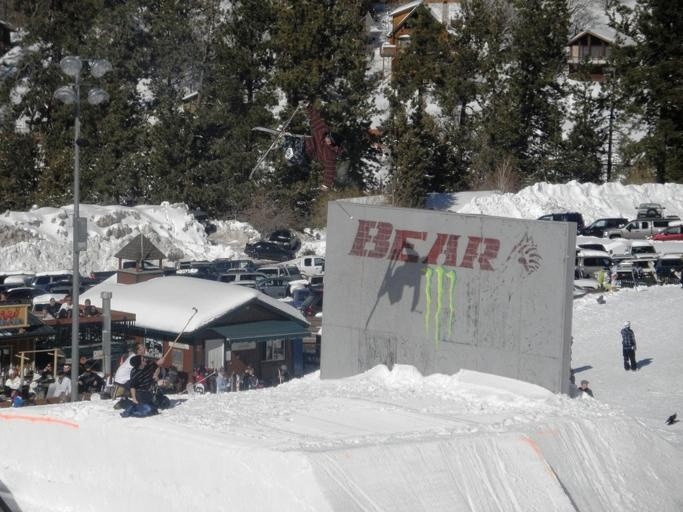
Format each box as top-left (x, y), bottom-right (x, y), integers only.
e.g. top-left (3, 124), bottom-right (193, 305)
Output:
top-left (113, 399), bottom-right (124, 409)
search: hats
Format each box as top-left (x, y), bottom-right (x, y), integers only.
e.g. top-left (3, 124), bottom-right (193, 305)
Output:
top-left (243, 242), bottom-right (295, 263)
top-left (535, 203), bottom-right (683, 287)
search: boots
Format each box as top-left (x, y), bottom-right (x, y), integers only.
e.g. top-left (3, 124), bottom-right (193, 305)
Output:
top-left (269, 229), bottom-right (298, 250)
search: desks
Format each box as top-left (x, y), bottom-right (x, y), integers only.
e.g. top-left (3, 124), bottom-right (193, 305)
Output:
top-left (52, 52), bottom-right (115, 402)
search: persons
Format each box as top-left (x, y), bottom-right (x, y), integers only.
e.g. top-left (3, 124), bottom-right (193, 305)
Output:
top-left (278, 365), bottom-right (290, 384)
top-left (5, 339), bottom-right (261, 418)
top-left (578, 380), bottom-right (593, 397)
top-left (597, 267), bottom-right (605, 288)
top-left (42, 294), bottom-right (103, 320)
top-left (621, 321), bottom-right (636, 370)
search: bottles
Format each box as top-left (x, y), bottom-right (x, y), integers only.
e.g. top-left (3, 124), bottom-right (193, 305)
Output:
top-left (130, 355), bottom-right (141, 367)
